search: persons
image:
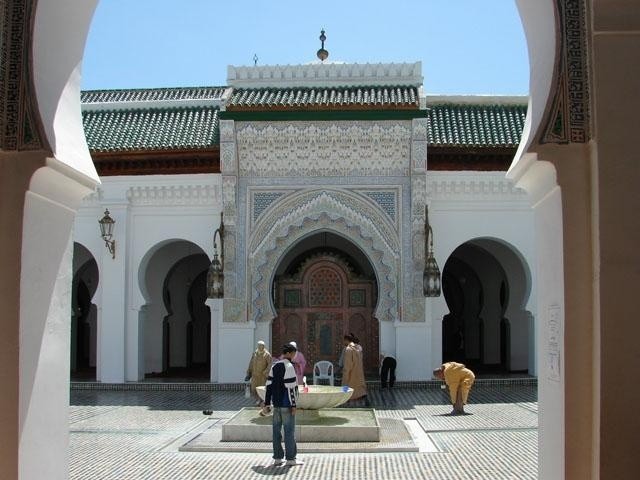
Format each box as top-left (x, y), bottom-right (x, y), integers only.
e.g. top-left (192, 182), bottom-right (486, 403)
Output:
top-left (278, 341), bottom-right (307, 385)
top-left (379, 351), bottom-right (397, 391)
top-left (263, 343), bottom-right (304, 465)
top-left (337, 337), bottom-right (363, 374)
top-left (341, 335), bottom-right (370, 406)
top-left (245, 340), bottom-right (272, 407)
top-left (432, 361), bottom-right (476, 416)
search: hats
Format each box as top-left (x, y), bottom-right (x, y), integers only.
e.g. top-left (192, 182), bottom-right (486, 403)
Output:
top-left (290, 342), bottom-right (297, 348)
top-left (258, 340), bottom-right (265, 346)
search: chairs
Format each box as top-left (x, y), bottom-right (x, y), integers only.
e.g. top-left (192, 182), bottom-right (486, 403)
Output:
top-left (313, 360), bottom-right (335, 385)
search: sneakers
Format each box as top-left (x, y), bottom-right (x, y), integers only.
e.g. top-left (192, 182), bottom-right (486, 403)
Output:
top-left (275, 458), bottom-right (286, 465)
top-left (256, 399), bottom-right (261, 406)
top-left (365, 400), bottom-right (369, 406)
top-left (455, 410), bottom-right (466, 416)
top-left (286, 459), bottom-right (304, 465)
